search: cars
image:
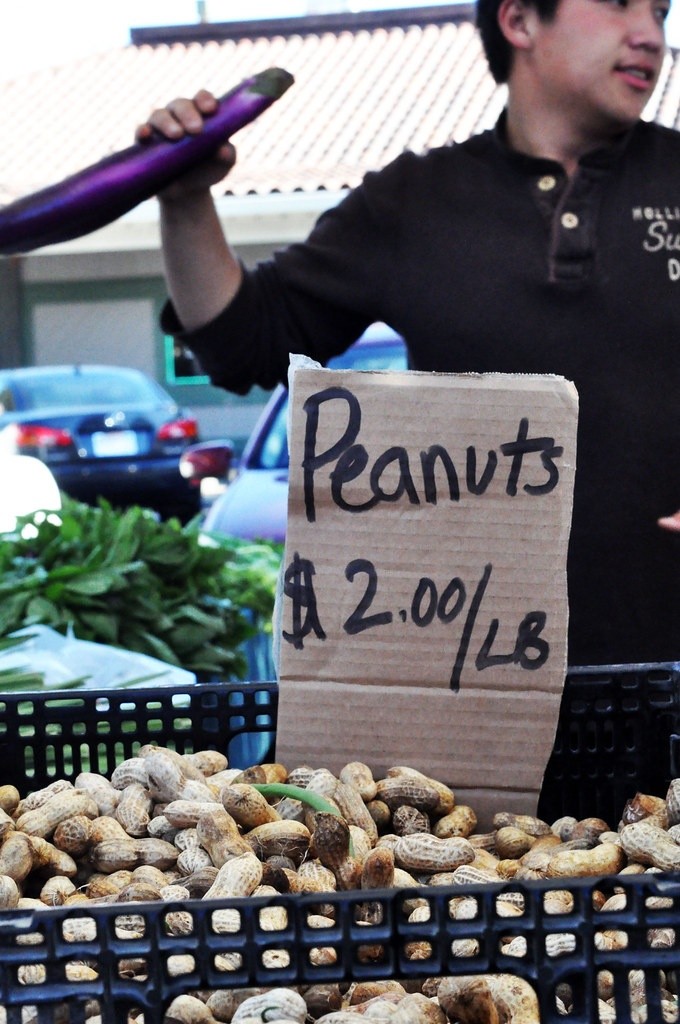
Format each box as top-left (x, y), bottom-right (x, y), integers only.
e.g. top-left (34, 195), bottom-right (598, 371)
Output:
top-left (180, 337), bottom-right (409, 570)
top-left (0, 362), bottom-right (235, 528)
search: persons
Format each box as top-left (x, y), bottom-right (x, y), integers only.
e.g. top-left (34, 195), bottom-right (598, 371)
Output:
top-left (134, 0), bottom-right (680, 815)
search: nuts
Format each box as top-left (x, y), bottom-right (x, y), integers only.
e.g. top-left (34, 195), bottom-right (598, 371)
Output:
top-left (0, 743), bottom-right (680, 1024)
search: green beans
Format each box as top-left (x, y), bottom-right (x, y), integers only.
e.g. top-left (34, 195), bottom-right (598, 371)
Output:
top-left (249, 779), bottom-right (354, 860)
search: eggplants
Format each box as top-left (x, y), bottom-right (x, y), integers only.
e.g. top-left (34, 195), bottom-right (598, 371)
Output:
top-left (0, 66), bottom-right (295, 256)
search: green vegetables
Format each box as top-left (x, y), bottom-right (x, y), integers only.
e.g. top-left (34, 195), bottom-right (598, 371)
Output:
top-left (0, 488), bottom-right (285, 779)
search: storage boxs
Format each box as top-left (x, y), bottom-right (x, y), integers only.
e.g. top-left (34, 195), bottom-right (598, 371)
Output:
top-left (0, 660), bottom-right (680, 1024)
top-left (0, 602), bottom-right (277, 768)
